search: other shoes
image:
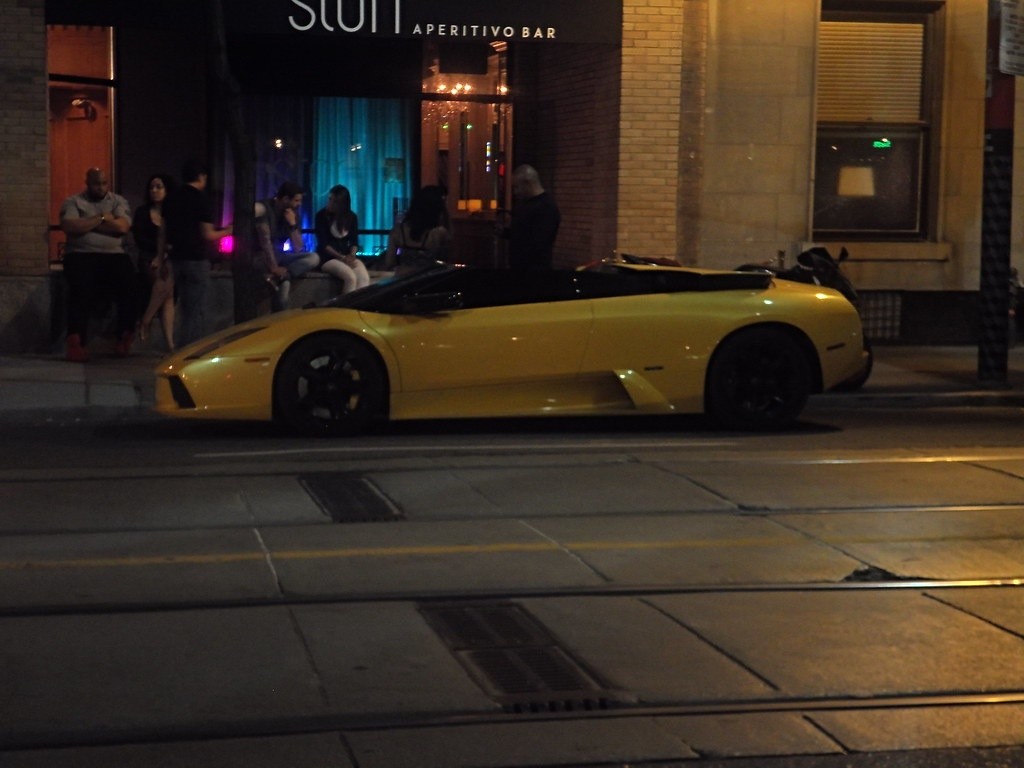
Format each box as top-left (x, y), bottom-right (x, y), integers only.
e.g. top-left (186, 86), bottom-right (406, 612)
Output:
top-left (115, 329), bottom-right (137, 357)
top-left (139, 324), bottom-right (145, 341)
top-left (65, 333), bottom-right (90, 361)
top-left (265, 274), bottom-right (279, 292)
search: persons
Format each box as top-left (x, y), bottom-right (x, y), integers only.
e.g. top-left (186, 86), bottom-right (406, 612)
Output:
top-left (314, 185), bottom-right (371, 294)
top-left (132, 175), bottom-right (175, 350)
top-left (157, 159), bottom-right (232, 349)
top-left (232, 159), bottom-right (278, 324)
top-left (385, 186), bottom-right (452, 279)
top-left (261, 181), bottom-right (321, 307)
top-left (494, 164), bottom-right (562, 271)
top-left (60, 167), bottom-right (133, 362)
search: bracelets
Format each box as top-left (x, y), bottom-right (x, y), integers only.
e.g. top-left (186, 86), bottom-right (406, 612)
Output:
top-left (289, 224), bottom-right (299, 231)
top-left (95, 215), bottom-right (99, 224)
top-left (101, 213), bottom-right (105, 224)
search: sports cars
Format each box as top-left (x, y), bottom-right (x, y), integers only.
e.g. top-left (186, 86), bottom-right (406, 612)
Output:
top-left (148, 248), bottom-right (875, 443)
